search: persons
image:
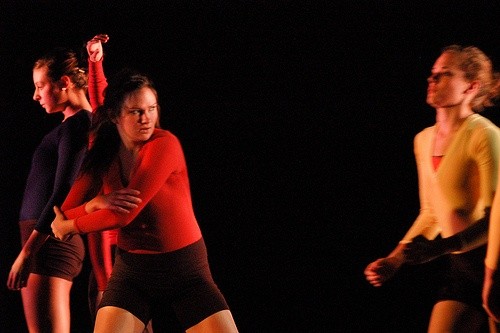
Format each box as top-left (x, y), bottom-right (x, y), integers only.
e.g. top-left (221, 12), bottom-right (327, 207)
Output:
top-left (6, 53), bottom-right (93, 333)
top-left (50, 75), bottom-right (237, 332)
top-left (364, 44), bottom-right (500, 333)
top-left (86, 33), bottom-right (153, 332)
